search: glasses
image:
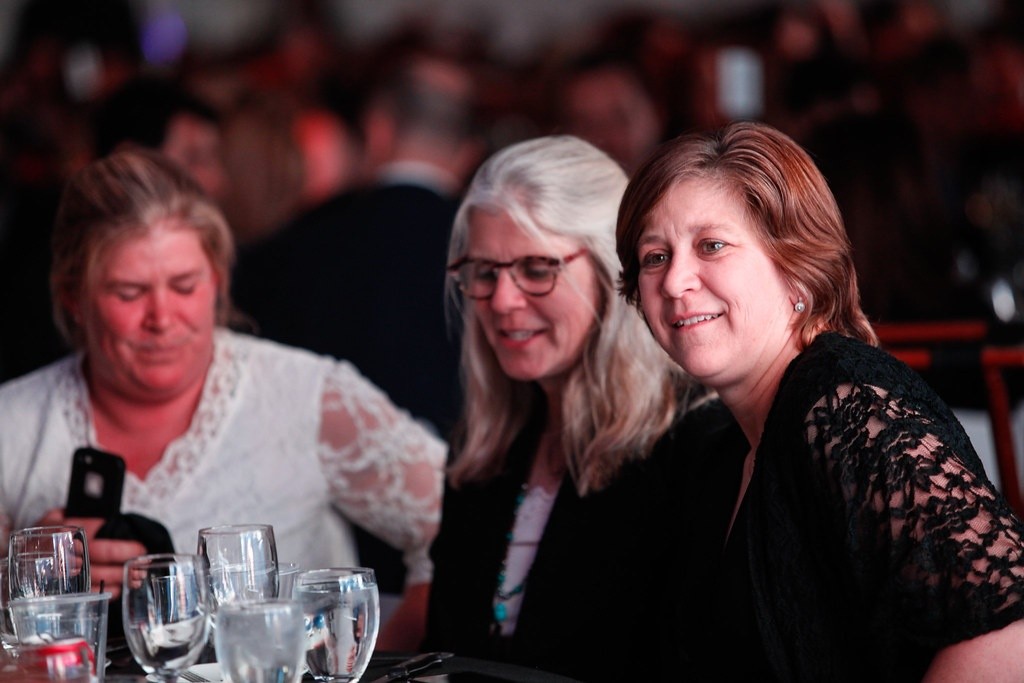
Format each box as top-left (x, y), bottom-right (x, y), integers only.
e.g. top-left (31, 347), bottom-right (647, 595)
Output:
top-left (448, 248), bottom-right (586, 300)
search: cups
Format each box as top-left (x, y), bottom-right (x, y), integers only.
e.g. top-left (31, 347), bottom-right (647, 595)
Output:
top-left (0, 552), bottom-right (58, 652)
top-left (8, 591), bottom-right (111, 679)
top-left (198, 523), bottom-right (381, 683)
top-left (122, 555), bottom-right (211, 682)
top-left (8, 525), bottom-right (92, 600)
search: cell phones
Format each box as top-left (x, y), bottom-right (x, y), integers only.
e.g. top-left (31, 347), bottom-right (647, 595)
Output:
top-left (61, 448), bottom-right (126, 542)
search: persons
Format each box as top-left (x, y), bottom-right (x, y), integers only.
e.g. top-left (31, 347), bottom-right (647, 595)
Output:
top-left (0, 0), bottom-right (1024, 683)
